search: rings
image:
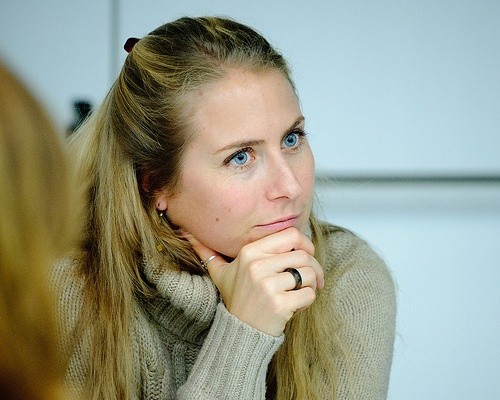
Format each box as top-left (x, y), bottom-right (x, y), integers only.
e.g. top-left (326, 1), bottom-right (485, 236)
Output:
top-left (284, 268), bottom-right (302, 290)
top-left (200, 253), bottom-right (219, 271)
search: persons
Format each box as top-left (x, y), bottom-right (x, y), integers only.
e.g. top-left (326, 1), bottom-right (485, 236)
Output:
top-left (0, 59), bottom-right (84, 400)
top-left (46, 16), bottom-right (397, 400)
